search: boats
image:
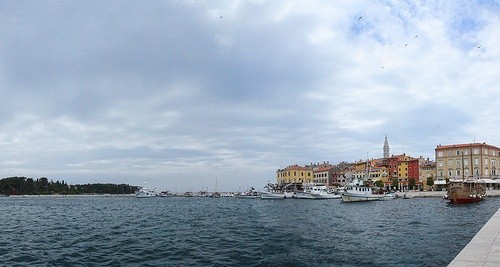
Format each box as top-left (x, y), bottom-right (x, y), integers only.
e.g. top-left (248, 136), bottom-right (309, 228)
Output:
top-left (339, 185), bottom-right (397, 202)
top-left (443, 181), bottom-right (486, 205)
top-left (292, 183), bottom-right (342, 199)
top-left (135, 178), bottom-right (293, 199)
